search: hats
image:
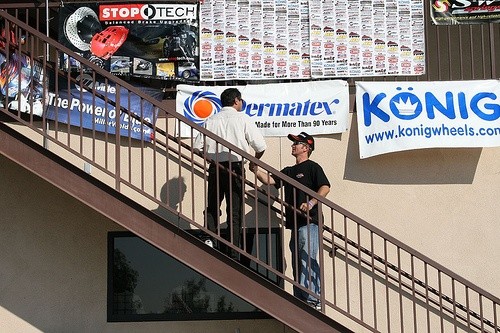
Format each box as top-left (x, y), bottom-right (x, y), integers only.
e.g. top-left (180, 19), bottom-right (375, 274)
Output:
top-left (288, 131), bottom-right (314, 151)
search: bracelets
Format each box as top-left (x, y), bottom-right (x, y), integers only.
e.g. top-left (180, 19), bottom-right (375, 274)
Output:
top-left (308, 200), bottom-right (315, 207)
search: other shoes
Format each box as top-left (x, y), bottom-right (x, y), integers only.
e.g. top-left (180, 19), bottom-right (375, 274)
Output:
top-left (205, 239), bottom-right (213, 248)
top-left (305, 301), bottom-right (322, 312)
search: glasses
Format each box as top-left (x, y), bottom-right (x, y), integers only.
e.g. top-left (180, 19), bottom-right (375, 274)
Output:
top-left (293, 140), bottom-right (308, 146)
top-left (239, 99), bottom-right (243, 103)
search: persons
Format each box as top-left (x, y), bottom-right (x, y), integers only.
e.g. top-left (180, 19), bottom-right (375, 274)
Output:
top-left (194, 88), bottom-right (266, 263)
top-left (249, 132), bottom-right (330, 311)
